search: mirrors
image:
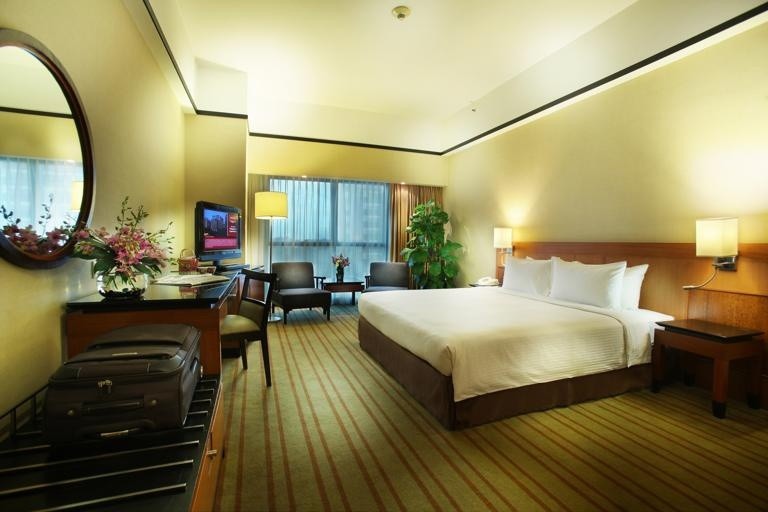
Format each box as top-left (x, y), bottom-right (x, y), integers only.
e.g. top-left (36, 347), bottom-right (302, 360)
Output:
top-left (0, 28), bottom-right (97, 272)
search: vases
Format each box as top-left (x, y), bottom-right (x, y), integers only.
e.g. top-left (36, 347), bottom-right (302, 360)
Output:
top-left (96, 270), bottom-right (149, 299)
top-left (335, 268), bottom-right (344, 281)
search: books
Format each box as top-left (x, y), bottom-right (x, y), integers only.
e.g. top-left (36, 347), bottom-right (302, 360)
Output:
top-left (154, 272), bottom-right (229, 286)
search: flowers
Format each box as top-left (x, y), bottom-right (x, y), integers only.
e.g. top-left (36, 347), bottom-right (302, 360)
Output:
top-left (0, 191), bottom-right (79, 256)
top-left (332, 255), bottom-right (350, 272)
top-left (69, 193), bottom-right (179, 287)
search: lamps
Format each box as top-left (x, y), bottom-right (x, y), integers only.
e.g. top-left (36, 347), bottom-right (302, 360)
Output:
top-left (70, 180), bottom-right (83, 211)
top-left (254, 191), bottom-right (289, 323)
top-left (493, 228), bottom-right (512, 269)
top-left (696, 215), bottom-right (739, 271)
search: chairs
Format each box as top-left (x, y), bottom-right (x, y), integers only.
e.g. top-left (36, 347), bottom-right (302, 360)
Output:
top-left (220, 267), bottom-right (279, 388)
top-left (363, 262), bottom-right (409, 294)
top-left (272, 262), bottom-right (332, 324)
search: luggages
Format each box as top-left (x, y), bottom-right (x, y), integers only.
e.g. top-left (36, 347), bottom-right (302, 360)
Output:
top-left (37, 316), bottom-right (207, 455)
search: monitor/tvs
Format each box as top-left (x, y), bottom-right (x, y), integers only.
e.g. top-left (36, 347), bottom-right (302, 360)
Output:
top-left (195, 200), bottom-right (242, 267)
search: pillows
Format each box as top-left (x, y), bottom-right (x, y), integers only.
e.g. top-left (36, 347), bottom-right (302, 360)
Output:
top-left (525, 256), bottom-right (535, 260)
top-left (549, 256), bottom-right (630, 312)
top-left (501, 255), bottom-right (561, 300)
top-left (623, 264), bottom-right (652, 312)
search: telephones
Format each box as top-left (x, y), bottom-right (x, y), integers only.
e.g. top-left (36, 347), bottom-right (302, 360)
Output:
top-left (478, 277), bottom-right (499, 285)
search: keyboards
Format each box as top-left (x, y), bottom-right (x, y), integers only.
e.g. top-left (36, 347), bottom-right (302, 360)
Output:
top-left (221, 264), bottom-right (251, 271)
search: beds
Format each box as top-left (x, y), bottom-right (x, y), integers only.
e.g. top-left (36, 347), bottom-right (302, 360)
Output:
top-left (354, 286), bottom-right (675, 428)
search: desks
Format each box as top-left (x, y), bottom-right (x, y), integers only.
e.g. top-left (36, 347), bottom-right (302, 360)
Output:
top-left (64, 272), bottom-right (241, 382)
top-left (469, 283), bottom-right (502, 288)
top-left (650, 317), bottom-right (764, 419)
top-left (320, 280), bottom-right (364, 315)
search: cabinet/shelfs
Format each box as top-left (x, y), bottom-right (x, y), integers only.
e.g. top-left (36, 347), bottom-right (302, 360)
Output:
top-left (223, 264), bottom-right (266, 315)
top-left (1, 376), bottom-right (228, 512)
